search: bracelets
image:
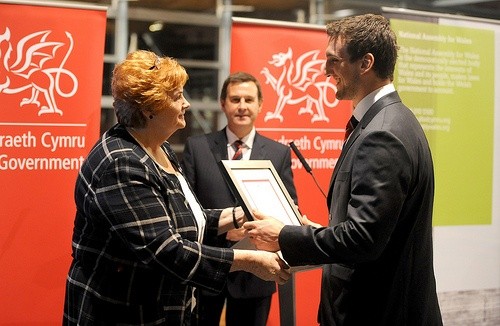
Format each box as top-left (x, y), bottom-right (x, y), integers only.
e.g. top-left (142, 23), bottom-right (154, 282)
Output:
top-left (232, 207), bottom-right (241, 229)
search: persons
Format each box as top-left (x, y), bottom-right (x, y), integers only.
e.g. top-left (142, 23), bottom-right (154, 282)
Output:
top-left (63, 49), bottom-right (291, 326)
top-left (243, 14), bottom-right (443, 326)
top-left (183, 71), bottom-right (300, 326)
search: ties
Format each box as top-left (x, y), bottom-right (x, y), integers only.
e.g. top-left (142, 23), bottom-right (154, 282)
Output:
top-left (341, 114), bottom-right (359, 148)
top-left (232, 140), bottom-right (244, 160)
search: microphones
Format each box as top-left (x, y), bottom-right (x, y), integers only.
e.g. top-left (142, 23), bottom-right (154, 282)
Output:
top-left (290, 142), bottom-right (327, 198)
